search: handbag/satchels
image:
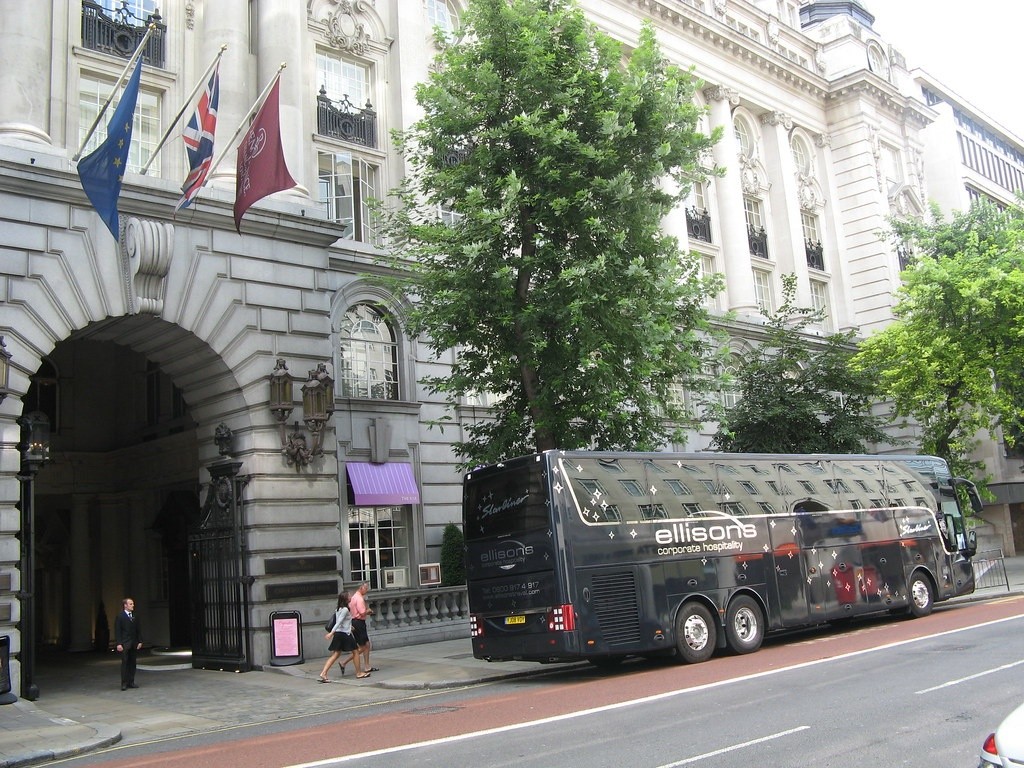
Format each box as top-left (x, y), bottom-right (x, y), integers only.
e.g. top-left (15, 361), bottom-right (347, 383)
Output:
top-left (325, 613), bottom-right (336, 633)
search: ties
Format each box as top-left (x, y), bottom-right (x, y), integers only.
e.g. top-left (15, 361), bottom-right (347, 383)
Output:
top-left (129, 613), bottom-right (131, 620)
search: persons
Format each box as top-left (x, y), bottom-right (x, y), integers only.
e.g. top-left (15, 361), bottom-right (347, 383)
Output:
top-left (317, 591), bottom-right (370, 683)
top-left (338, 580), bottom-right (379, 674)
top-left (114, 597), bottom-right (142, 690)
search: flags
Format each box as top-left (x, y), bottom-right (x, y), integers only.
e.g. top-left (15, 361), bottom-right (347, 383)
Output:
top-left (232, 76), bottom-right (296, 238)
top-left (78, 53), bottom-right (142, 241)
top-left (175, 63), bottom-right (219, 215)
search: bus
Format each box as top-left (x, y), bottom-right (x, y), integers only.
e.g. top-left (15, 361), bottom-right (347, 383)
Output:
top-left (461, 450), bottom-right (986, 671)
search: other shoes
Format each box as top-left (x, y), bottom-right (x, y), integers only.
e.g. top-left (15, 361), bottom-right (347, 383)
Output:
top-left (122, 685), bottom-right (126, 690)
top-left (128, 683), bottom-right (139, 688)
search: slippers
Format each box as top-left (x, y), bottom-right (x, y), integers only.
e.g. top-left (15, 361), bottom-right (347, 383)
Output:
top-left (365, 668), bottom-right (379, 672)
top-left (339, 662), bottom-right (345, 675)
top-left (317, 679), bottom-right (332, 683)
top-left (357, 672), bottom-right (370, 678)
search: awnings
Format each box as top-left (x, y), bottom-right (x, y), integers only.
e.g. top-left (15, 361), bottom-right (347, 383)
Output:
top-left (347, 462), bottom-right (420, 505)
